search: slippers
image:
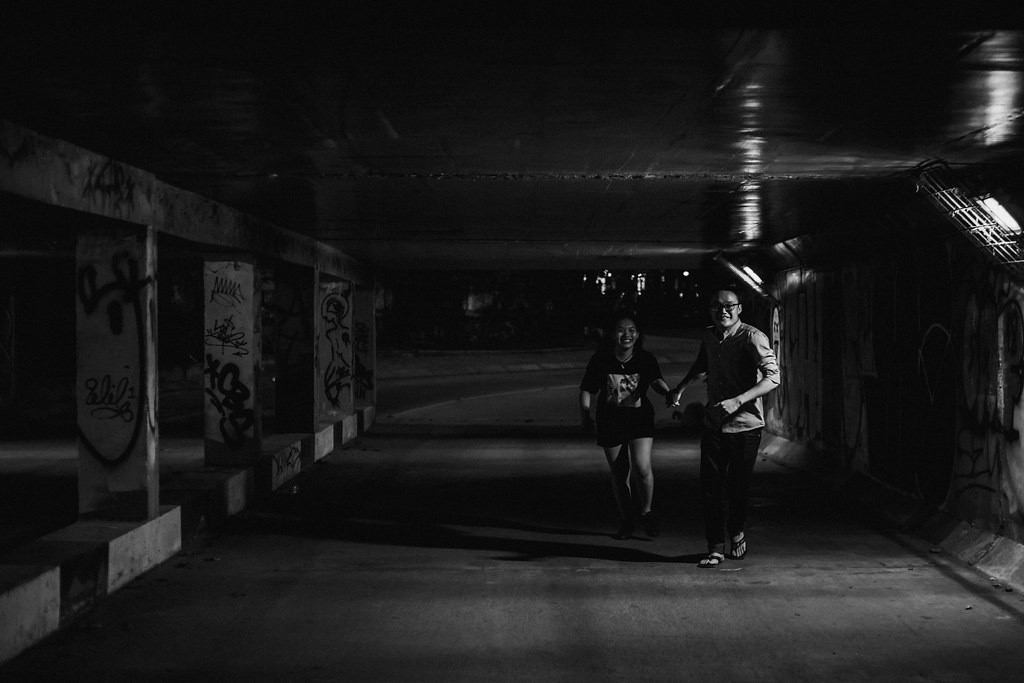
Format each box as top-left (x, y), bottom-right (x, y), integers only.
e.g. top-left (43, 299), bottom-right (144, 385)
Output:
top-left (697, 556), bottom-right (725, 568)
top-left (729, 536), bottom-right (747, 562)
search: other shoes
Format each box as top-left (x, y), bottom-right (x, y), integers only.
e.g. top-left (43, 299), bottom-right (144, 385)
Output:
top-left (616, 512), bottom-right (635, 540)
top-left (638, 507), bottom-right (659, 537)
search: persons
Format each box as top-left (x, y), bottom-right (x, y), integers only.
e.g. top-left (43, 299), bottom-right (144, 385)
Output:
top-left (666, 289), bottom-right (780, 567)
top-left (580, 313), bottom-right (680, 541)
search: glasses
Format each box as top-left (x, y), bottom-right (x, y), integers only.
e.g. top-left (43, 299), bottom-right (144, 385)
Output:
top-left (710, 303), bottom-right (739, 312)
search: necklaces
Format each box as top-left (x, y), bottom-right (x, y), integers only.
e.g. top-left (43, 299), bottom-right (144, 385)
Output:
top-left (614, 355), bottom-right (632, 369)
